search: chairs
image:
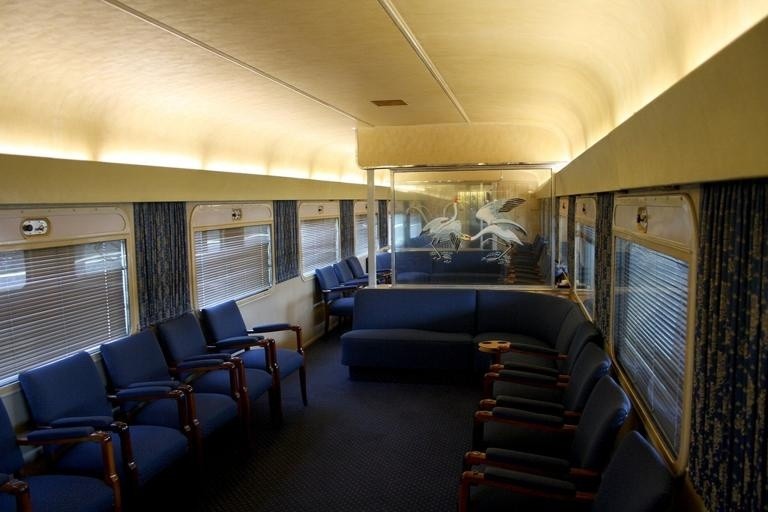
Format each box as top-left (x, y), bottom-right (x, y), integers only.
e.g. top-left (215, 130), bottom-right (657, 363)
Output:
top-left (507, 234), bottom-right (563, 285)
top-left (464, 374), bottom-right (632, 492)
top-left (19, 349), bottom-right (194, 506)
top-left (149, 309), bottom-right (277, 436)
top-left (1, 398), bottom-right (121, 511)
top-left (198, 299), bottom-right (308, 416)
top-left (459, 430), bottom-right (677, 511)
top-left (99, 327), bottom-right (244, 479)
top-left (477, 342), bottom-right (612, 425)
top-left (474, 320), bottom-right (599, 382)
top-left (318, 257), bottom-right (392, 336)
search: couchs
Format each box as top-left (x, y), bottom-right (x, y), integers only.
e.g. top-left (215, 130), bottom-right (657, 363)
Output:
top-left (341, 288), bottom-right (588, 382)
top-left (365, 250), bottom-right (505, 285)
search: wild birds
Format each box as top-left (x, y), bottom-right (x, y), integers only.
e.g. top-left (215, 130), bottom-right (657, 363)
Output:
top-left (405, 194), bottom-right (528, 263)
